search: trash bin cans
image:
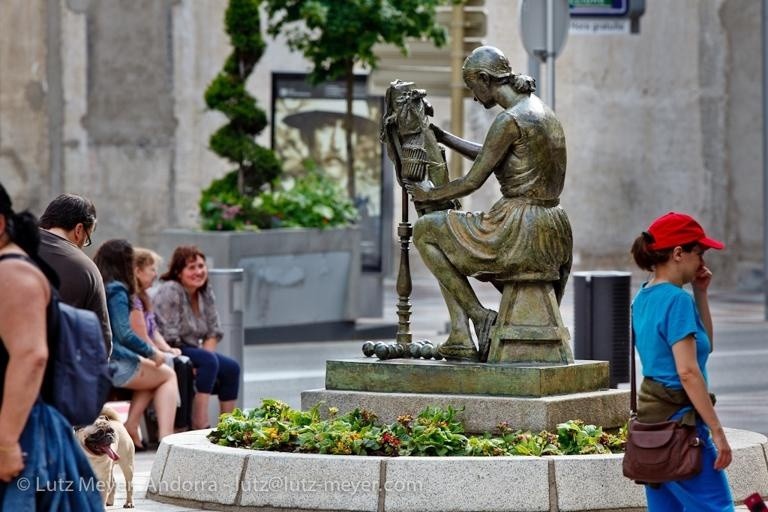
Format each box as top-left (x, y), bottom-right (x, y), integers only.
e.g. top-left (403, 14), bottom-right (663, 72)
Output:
top-left (191, 267), bottom-right (245, 429)
top-left (572, 270), bottom-right (630, 389)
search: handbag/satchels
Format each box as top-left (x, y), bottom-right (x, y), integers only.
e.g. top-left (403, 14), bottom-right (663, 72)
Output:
top-left (622, 420), bottom-right (702, 484)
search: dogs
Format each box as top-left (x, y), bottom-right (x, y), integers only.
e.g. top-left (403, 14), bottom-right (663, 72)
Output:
top-left (73, 406), bottom-right (137, 510)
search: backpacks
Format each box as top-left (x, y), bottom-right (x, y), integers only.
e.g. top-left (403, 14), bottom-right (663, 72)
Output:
top-left (1, 253), bottom-right (115, 427)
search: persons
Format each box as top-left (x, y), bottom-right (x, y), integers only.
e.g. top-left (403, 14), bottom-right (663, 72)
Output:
top-left (281, 99), bottom-right (381, 220)
top-left (128, 246), bottom-right (195, 447)
top-left (0, 182), bottom-right (105, 510)
top-left (37, 191), bottom-right (115, 363)
top-left (93, 238), bottom-right (179, 452)
top-left (151, 242), bottom-right (243, 433)
top-left (628, 211), bottom-right (736, 512)
top-left (412, 46), bottom-right (575, 364)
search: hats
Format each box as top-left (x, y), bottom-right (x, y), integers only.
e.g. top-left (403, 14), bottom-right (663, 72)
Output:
top-left (645, 213), bottom-right (724, 250)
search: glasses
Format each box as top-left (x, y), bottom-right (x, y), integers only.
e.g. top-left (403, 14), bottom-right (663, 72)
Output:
top-left (83, 227), bottom-right (91, 247)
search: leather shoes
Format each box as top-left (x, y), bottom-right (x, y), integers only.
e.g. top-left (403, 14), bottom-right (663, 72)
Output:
top-left (135, 440), bottom-right (147, 451)
